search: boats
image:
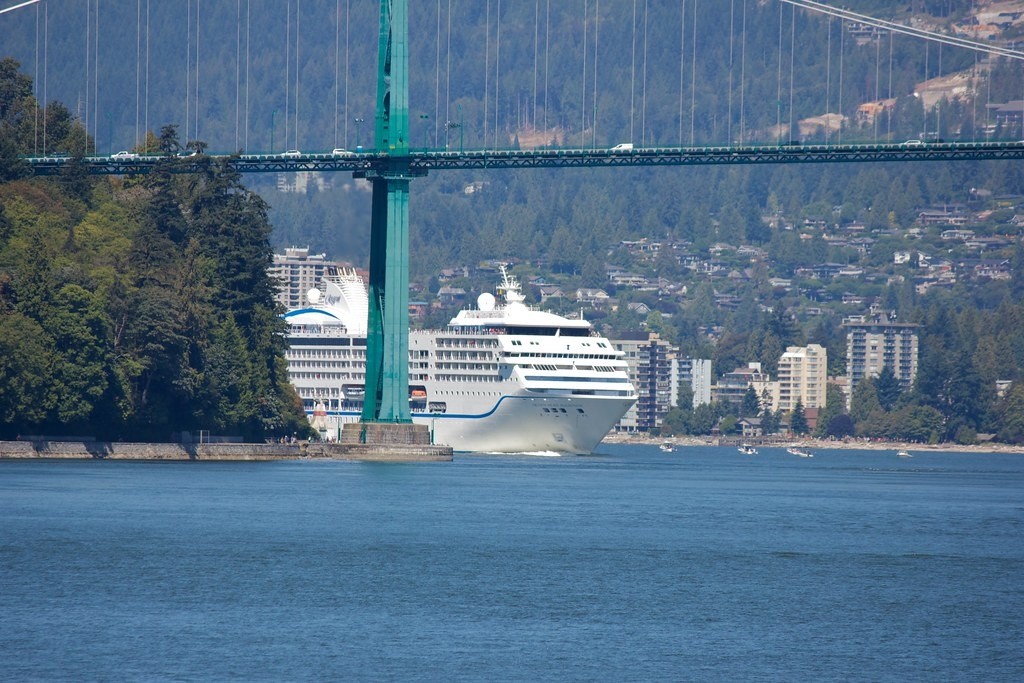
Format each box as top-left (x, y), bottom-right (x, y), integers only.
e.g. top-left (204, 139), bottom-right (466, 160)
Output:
top-left (896, 451), bottom-right (912, 458)
top-left (275, 265), bottom-right (642, 454)
top-left (659, 440), bottom-right (678, 453)
top-left (787, 448), bottom-right (814, 458)
top-left (738, 444), bottom-right (759, 455)
